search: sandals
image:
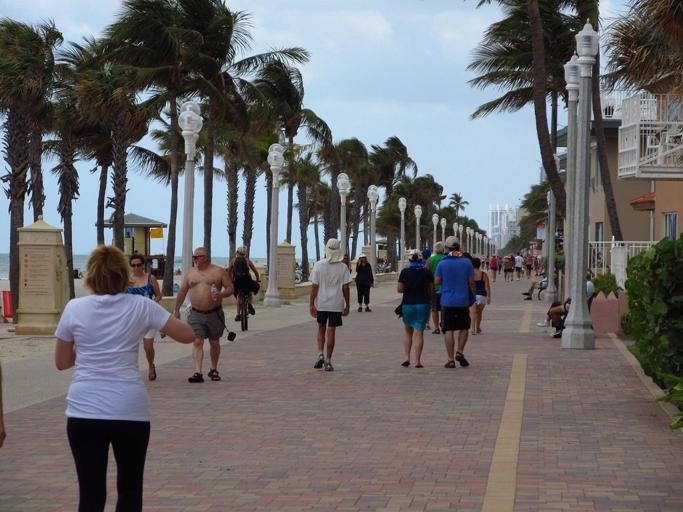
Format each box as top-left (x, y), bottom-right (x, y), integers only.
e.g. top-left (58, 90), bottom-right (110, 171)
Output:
top-left (187, 373), bottom-right (203, 383)
top-left (149, 364), bottom-right (156, 380)
top-left (431, 329), bottom-right (439, 334)
top-left (208, 369), bottom-right (220, 381)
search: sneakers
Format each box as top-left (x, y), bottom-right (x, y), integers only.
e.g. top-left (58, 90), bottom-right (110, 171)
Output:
top-left (536, 321), bottom-right (549, 326)
top-left (325, 363), bottom-right (332, 371)
top-left (444, 361), bottom-right (455, 368)
top-left (455, 352), bottom-right (468, 367)
top-left (313, 354), bottom-right (323, 368)
top-left (424, 324), bottom-right (430, 330)
top-left (365, 307), bottom-right (370, 312)
top-left (358, 308), bottom-right (361, 312)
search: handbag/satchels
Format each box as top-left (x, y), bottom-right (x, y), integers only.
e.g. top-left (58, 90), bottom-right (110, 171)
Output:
top-left (394, 304), bottom-right (401, 318)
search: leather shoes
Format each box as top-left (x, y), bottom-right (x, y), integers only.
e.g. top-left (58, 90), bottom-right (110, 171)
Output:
top-left (553, 332), bottom-right (560, 338)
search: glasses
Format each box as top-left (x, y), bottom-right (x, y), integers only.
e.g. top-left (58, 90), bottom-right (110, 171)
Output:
top-left (130, 263), bottom-right (141, 267)
top-left (192, 255), bottom-right (205, 259)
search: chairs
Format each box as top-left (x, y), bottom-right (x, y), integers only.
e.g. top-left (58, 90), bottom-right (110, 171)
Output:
top-left (645, 124), bottom-right (682, 165)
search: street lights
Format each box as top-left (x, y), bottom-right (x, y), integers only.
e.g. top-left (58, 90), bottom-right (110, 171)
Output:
top-left (517, 240), bottom-right (538, 259)
top-left (542, 152), bottom-right (561, 305)
top-left (432, 213), bottom-right (499, 274)
top-left (414, 204), bottom-right (423, 251)
top-left (397, 196), bottom-right (406, 281)
top-left (178, 101), bottom-right (203, 323)
top-left (547, 190), bottom-right (551, 283)
top-left (336, 173), bottom-right (351, 265)
top-left (366, 185), bottom-right (379, 288)
top-left (263, 142), bottom-right (284, 308)
top-left (562, 17), bottom-right (601, 350)
top-left (563, 50), bottom-right (581, 321)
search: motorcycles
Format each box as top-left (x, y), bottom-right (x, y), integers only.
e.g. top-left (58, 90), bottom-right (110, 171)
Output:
top-left (295, 262), bottom-right (303, 285)
top-left (377, 256), bottom-right (393, 273)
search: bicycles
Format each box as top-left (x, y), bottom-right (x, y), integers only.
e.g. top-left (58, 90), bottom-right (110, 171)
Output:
top-left (231, 280), bottom-right (261, 331)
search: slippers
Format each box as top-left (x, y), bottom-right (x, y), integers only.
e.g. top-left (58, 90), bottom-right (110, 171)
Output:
top-left (415, 365), bottom-right (424, 368)
top-left (401, 362), bottom-right (409, 366)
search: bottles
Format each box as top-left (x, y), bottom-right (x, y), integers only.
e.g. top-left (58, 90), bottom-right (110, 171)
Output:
top-left (210, 283), bottom-right (218, 302)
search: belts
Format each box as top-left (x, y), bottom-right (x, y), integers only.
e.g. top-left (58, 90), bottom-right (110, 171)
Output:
top-left (192, 305), bottom-right (221, 314)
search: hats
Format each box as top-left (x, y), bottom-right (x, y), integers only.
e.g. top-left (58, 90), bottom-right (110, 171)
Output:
top-left (445, 236), bottom-right (459, 249)
top-left (325, 238), bottom-right (344, 263)
top-left (408, 249), bottom-right (422, 260)
top-left (358, 253), bottom-right (366, 258)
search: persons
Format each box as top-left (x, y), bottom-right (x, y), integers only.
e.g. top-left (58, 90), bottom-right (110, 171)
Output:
top-left (342, 242), bottom-right (594, 338)
top-left (308, 237), bottom-right (352, 370)
top-left (173, 247), bottom-right (235, 381)
top-left (434, 235), bottom-right (476, 367)
top-left (51, 244), bottom-right (194, 511)
top-left (226, 246), bottom-right (260, 321)
top-left (122, 253), bottom-right (162, 381)
top-left (396, 249), bottom-right (434, 368)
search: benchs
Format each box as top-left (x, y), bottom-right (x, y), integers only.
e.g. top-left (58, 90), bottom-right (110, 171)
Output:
top-left (537, 276), bottom-right (559, 301)
top-left (556, 290), bottom-right (594, 338)
